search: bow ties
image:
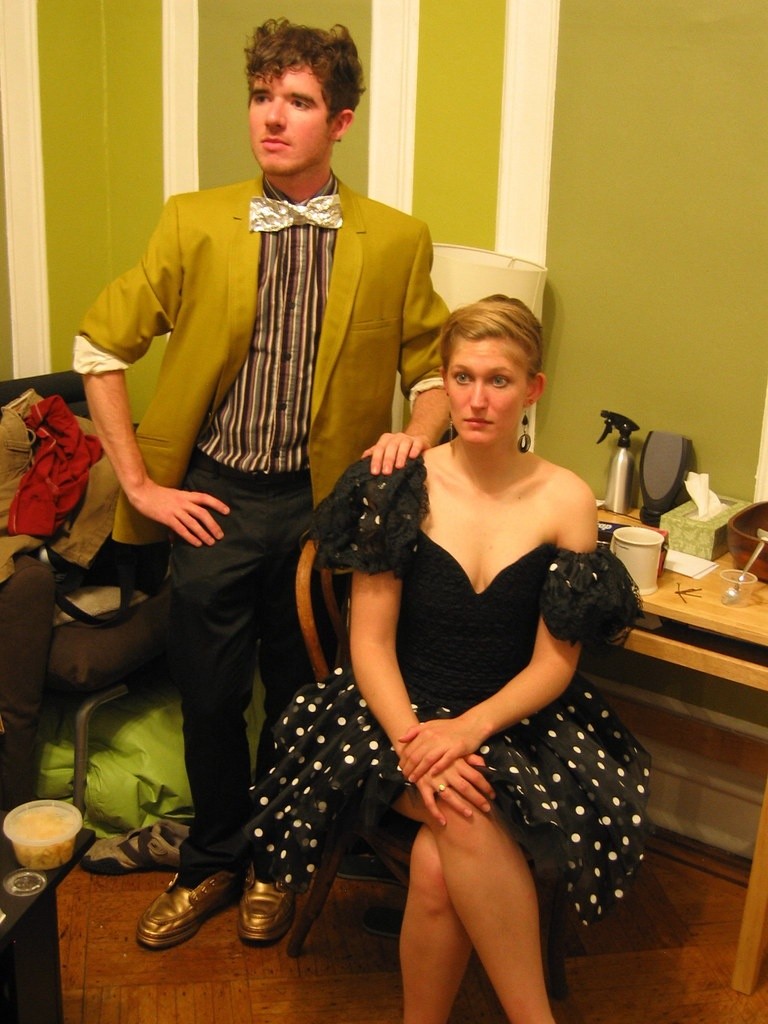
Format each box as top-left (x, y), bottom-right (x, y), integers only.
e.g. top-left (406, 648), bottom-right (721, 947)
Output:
top-left (249, 194), bottom-right (344, 231)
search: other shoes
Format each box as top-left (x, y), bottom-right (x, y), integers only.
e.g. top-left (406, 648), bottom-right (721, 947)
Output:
top-left (362, 907), bottom-right (404, 939)
top-left (336, 850), bottom-right (410, 883)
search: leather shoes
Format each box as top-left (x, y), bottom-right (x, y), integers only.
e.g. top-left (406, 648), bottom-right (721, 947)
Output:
top-left (240, 858), bottom-right (297, 943)
top-left (137, 871), bottom-right (239, 946)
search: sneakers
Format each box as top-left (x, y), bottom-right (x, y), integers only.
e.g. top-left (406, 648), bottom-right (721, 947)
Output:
top-left (79, 818), bottom-right (191, 876)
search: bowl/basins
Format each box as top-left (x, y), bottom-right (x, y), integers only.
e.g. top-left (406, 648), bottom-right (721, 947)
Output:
top-left (2, 800), bottom-right (82, 870)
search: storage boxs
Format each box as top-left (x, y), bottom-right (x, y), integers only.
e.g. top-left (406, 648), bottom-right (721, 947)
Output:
top-left (660, 494), bottom-right (753, 562)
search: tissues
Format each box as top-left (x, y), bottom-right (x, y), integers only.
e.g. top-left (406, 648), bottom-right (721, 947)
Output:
top-left (659, 472), bottom-right (754, 562)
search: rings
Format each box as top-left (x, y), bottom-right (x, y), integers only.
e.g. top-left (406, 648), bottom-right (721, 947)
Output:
top-left (438, 784), bottom-right (448, 795)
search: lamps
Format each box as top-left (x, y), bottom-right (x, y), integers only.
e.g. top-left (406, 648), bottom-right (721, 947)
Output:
top-left (427, 242), bottom-right (548, 327)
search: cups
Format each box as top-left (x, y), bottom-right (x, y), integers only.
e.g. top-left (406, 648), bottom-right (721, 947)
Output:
top-left (720, 569), bottom-right (758, 608)
top-left (610, 527), bottom-right (664, 595)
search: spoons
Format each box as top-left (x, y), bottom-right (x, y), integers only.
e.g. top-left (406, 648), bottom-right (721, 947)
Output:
top-left (723, 541), bottom-right (765, 604)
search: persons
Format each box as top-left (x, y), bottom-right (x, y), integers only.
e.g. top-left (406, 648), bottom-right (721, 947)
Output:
top-left (74, 20), bottom-right (451, 950)
top-left (350, 293), bottom-right (599, 1024)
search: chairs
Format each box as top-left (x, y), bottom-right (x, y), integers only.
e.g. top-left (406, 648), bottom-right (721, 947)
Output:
top-left (287, 508), bottom-right (415, 962)
top-left (0, 372), bottom-right (171, 819)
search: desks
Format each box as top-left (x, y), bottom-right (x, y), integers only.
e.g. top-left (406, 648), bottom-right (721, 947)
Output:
top-left (594, 500), bottom-right (768, 995)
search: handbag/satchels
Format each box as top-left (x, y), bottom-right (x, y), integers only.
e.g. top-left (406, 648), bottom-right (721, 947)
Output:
top-left (38, 541), bottom-right (168, 625)
